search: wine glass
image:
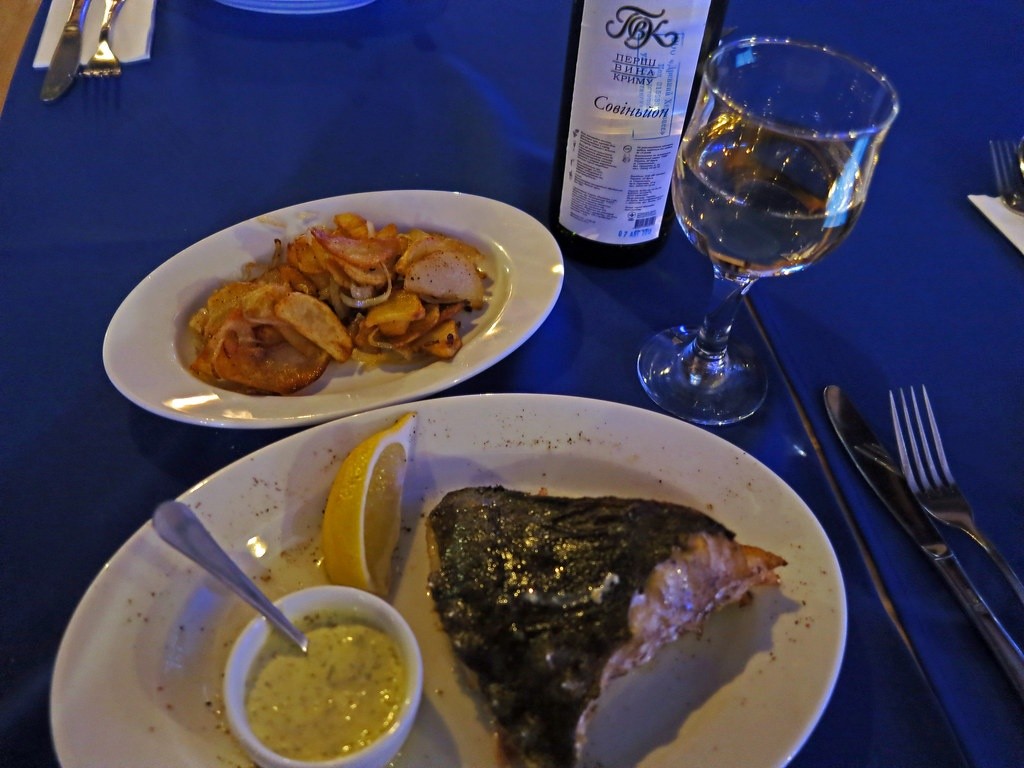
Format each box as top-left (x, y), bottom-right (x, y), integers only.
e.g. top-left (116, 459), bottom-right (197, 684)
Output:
top-left (636, 35), bottom-right (898, 426)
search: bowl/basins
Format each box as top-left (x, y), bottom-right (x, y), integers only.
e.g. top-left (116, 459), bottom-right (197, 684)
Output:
top-left (222, 584), bottom-right (424, 768)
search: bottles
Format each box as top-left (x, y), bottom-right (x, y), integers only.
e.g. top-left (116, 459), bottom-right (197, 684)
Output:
top-left (552, 0), bottom-right (729, 270)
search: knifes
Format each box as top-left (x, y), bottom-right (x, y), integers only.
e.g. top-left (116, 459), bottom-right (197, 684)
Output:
top-left (40, 0), bottom-right (92, 102)
top-left (824, 385), bottom-right (1024, 702)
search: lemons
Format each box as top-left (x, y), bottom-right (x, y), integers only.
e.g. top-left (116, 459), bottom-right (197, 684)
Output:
top-left (325, 412), bottom-right (416, 597)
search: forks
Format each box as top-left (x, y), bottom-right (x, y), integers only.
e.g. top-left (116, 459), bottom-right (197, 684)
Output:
top-left (989, 139), bottom-right (1024, 217)
top-left (68, 0), bottom-right (125, 78)
top-left (889, 385), bottom-right (1024, 608)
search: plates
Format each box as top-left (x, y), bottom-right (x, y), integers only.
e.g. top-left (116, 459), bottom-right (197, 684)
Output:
top-left (51, 393), bottom-right (847, 768)
top-left (104, 190), bottom-right (564, 429)
